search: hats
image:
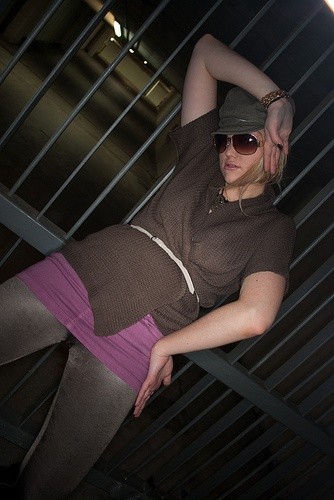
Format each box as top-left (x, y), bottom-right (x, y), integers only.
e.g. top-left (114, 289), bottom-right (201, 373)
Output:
top-left (216, 87), bottom-right (269, 134)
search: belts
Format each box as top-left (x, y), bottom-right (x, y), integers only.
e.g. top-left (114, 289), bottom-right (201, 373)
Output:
top-left (132, 222), bottom-right (200, 304)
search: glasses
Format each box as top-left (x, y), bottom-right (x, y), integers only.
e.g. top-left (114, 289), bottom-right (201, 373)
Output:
top-left (212, 133), bottom-right (264, 155)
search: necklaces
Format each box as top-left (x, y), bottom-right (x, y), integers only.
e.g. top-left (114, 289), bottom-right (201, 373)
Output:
top-left (208, 184), bottom-right (227, 215)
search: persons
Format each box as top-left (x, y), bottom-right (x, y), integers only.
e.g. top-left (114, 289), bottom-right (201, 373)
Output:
top-left (0, 34), bottom-right (294, 500)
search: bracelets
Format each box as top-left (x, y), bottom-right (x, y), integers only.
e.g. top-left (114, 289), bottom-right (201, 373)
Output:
top-left (260, 89), bottom-right (290, 109)
